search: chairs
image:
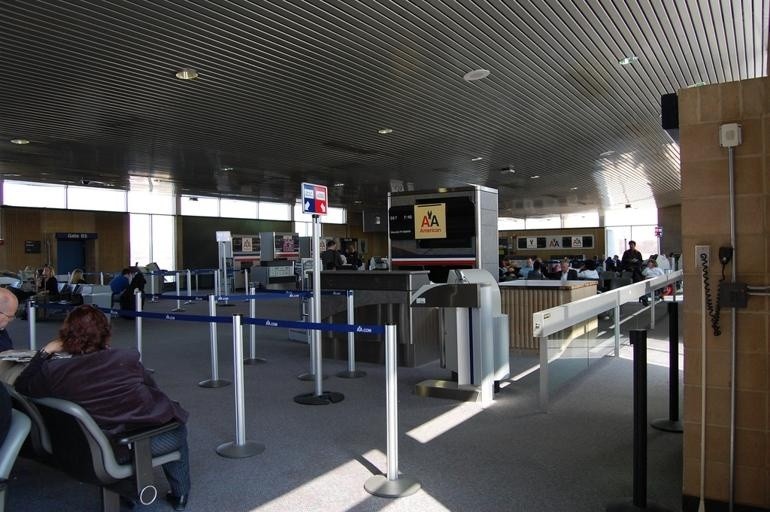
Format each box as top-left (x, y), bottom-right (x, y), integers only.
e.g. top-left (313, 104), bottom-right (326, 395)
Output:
top-left (1, 270), bottom-right (125, 318)
top-left (0, 369), bottom-right (183, 512)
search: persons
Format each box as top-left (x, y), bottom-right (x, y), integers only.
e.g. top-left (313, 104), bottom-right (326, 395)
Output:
top-left (0, 286), bottom-right (18, 353)
top-left (13, 303), bottom-right (192, 511)
top-left (106, 268), bottom-right (131, 294)
top-left (20, 264), bottom-right (61, 320)
top-left (320, 241), bottom-right (344, 271)
top-left (499, 241), bottom-right (672, 320)
top-left (67, 268), bottom-right (87, 284)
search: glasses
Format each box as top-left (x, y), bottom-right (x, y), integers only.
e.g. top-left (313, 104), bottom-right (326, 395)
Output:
top-left (0, 311), bottom-right (17, 324)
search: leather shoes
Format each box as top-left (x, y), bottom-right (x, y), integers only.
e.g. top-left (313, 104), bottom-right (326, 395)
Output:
top-left (166, 490), bottom-right (190, 512)
top-left (119, 494), bottom-right (138, 511)
